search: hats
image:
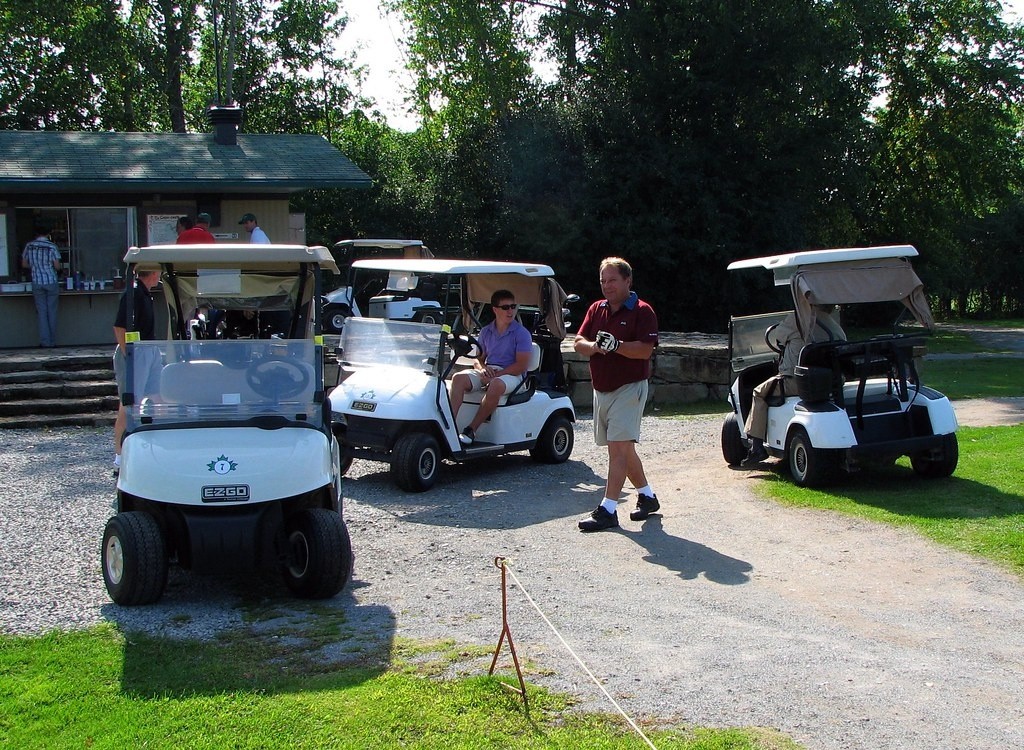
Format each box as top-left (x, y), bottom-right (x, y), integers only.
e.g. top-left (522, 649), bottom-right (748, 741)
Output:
top-left (134, 262), bottom-right (162, 271)
top-left (199, 213), bottom-right (212, 222)
top-left (238, 213), bottom-right (257, 224)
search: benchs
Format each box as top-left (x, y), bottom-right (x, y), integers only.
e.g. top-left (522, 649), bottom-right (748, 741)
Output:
top-left (794, 334), bottom-right (920, 411)
top-left (450, 335), bottom-right (544, 405)
top-left (159, 360), bottom-right (316, 406)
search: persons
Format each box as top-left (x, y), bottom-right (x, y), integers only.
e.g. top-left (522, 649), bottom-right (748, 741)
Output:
top-left (573, 257), bottom-right (660, 530)
top-left (740, 304), bottom-right (848, 467)
top-left (448, 290), bottom-right (533, 444)
top-left (112, 262), bottom-right (162, 475)
top-left (238, 214), bottom-right (270, 245)
top-left (21, 227), bottom-right (61, 346)
top-left (176, 212), bottom-right (216, 245)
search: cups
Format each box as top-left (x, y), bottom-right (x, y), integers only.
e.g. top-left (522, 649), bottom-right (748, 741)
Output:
top-left (67, 278), bottom-right (73, 289)
top-left (90, 281), bottom-right (96, 290)
top-left (100, 281), bottom-right (105, 290)
top-left (84, 281), bottom-right (89, 290)
top-left (113, 276), bottom-right (122, 289)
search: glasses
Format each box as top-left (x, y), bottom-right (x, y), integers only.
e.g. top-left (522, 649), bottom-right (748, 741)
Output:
top-left (495, 303), bottom-right (518, 311)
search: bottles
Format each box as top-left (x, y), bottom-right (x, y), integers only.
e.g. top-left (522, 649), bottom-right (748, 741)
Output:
top-left (76, 271), bottom-right (81, 290)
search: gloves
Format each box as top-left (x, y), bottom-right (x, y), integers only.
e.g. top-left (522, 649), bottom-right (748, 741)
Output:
top-left (596, 331), bottom-right (620, 355)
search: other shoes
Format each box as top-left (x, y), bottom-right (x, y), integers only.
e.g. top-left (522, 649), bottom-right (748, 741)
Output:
top-left (113, 462), bottom-right (120, 476)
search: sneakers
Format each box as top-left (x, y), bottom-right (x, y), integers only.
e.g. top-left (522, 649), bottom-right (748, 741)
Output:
top-left (628, 492), bottom-right (661, 521)
top-left (576, 505), bottom-right (619, 530)
top-left (460, 426), bottom-right (476, 445)
top-left (740, 447), bottom-right (770, 467)
